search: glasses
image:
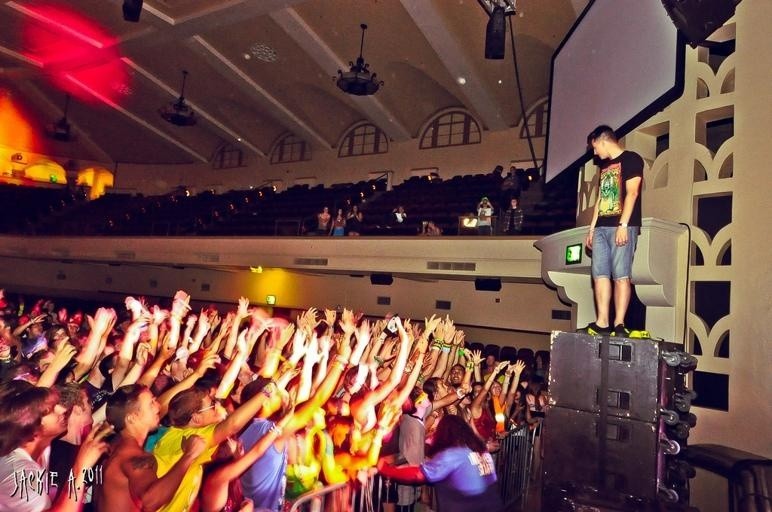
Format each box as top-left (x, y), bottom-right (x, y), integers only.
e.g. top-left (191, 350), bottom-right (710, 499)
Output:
top-left (189, 398), bottom-right (215, 418)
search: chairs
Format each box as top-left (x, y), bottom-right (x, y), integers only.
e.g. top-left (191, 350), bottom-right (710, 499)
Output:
top-left (24, 168), bottom-right (575, 235)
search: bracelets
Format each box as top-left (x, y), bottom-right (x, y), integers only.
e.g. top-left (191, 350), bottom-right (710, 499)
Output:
top-left (589, 229), bottom-right (594, 232)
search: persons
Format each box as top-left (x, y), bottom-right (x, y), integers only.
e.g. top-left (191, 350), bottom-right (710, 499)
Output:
top-left (502, 197), bottom-right (524, 235)
top-left (500, 166), bottom-right (519, 190)
top-left (417, 198), bottom-right (495, 236)
top-left (317, 205), bottom-right (363, 236)
top-left (489, 165), bottom-right (504, 181)
top-left (0, 289), bottom-right (545, 511)
top-left (392, 206), bottom-right (407, 224)
top-left (575, 124), bottom-right (644, 337)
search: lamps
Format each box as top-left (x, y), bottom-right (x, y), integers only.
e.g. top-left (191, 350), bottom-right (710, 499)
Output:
top-left (336, 22), bottom-right (384, 95)
top-left (43, 95), bottom-right (72, 141)
top-left (157, 70), bottom-right (196, 126)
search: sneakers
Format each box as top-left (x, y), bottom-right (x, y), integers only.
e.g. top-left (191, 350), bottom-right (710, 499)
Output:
top-left (575, 321), bottom-right (630, 338)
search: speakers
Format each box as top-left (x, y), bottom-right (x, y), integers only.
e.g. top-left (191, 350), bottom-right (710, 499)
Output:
top-left (539, 406), bottom-right (696, 511)
top-left (549, 329), bottom-right (686, 421)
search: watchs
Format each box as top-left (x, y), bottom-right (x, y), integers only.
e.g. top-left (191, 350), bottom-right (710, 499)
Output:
top-left (618, 222), bottom-right (628, 228)
